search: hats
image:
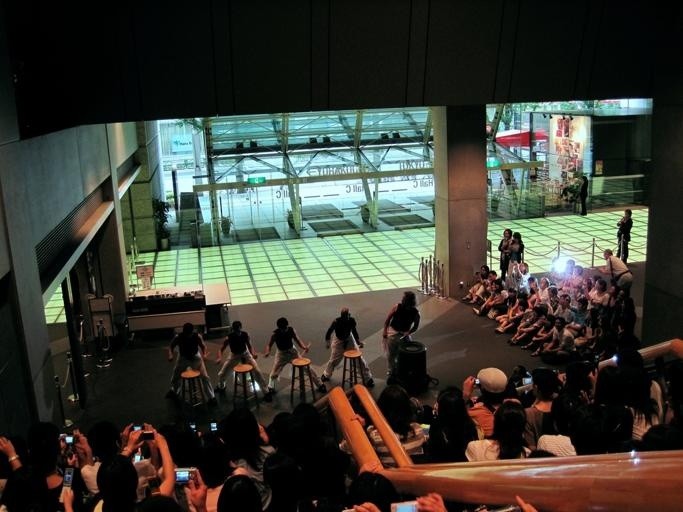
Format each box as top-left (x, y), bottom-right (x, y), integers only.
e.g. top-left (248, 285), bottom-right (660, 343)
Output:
top-left (477, 367), bottom-right (508, 392)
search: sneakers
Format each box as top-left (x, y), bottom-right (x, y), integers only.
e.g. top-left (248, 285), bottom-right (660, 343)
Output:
top-left (165, 374), bottom-right (375, 410)
top-left (462, 297), bottom-right (540, 357)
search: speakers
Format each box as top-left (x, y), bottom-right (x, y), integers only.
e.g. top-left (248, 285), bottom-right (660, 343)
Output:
top-left (203, 304), bottom-right (223, 336)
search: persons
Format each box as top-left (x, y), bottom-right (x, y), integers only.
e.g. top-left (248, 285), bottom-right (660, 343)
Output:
top-left (319, 307), bottom-right (376, 390)
top-left (463, 228), bottom-right (683, 512)
top-left (212, 321), bottom-right (274, 400)
top-left (164, 323), bottom-right (219, 406)
top-left (263, 316), bottom-right (327, 394)
top-left (1, 385), bottom-right (462, 512)
top-left (617, 209), bottom-right (635, 263)
top-left (381, 291), bottom-right (422, 377)
top-left (581, 173), bottom-right (590, 214)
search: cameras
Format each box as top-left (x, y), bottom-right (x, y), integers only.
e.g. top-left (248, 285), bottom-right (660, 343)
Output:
top-left (64, 436), bottom-right (77, 446)
top-left (474, 379), bottom-right (480, 384)
top-left (496, 505), bottom-right (521, 512)
top-left (174, 468), bottom-right (189, 484)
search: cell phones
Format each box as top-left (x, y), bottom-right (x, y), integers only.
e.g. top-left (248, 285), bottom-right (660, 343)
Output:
top-left (133, 425), bottom-right (144, 431)
top-left (390, 501), bottom-right (419, 512)
top-left (140, 432), bottom-right (154, 441)
top-left (209, 420), bottom-right (219, 431)
top-left (523, 377), bottom-right (533, 394)
top-left (134, 453), bottom-right (141, 463)
top-left (189, 422), bottom-right (196, 432)
top-left (59, 467), bottom-right (74, 504)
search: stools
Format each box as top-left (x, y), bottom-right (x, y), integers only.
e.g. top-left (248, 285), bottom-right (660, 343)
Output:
top-left (180, 350), bottom-right (366, 414)
